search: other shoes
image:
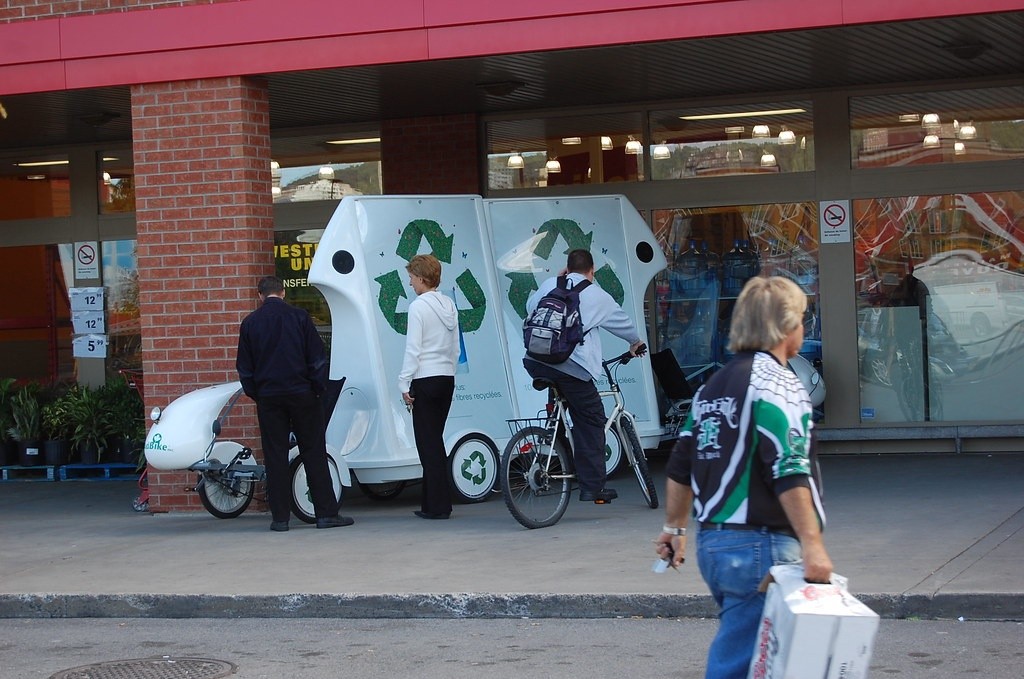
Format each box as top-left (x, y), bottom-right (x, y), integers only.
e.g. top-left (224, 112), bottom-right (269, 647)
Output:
top-left (545, 436), bottom-right (569, 448)
top-left (580, 489), bottom-right (618, 502)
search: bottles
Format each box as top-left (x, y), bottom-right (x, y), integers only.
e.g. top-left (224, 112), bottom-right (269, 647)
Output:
top-left (666, 236), bottom-right (819, 299)
top-left (665, 306), bottom-right (814, 361)
top-left (686, 371), bottom-right (708, 394)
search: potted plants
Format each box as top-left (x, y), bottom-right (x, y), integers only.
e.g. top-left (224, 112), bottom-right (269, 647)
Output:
top-left (0, 376), bottom-right (17, 466)
top-left (72, 385), bottom-right (111, 464)
top-left (13, 391), bottom-right (39, 467)
top-left (115, 392), bottom-right (139, 464)
top-left (41, 396), bottom-right (68, 465)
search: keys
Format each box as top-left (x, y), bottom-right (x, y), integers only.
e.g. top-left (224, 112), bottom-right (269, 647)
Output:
top-left (652, 539), bottom-right (686, 576)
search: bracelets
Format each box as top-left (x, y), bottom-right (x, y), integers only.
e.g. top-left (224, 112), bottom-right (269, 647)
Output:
top-left (662, 525), bottom-right (686, 536)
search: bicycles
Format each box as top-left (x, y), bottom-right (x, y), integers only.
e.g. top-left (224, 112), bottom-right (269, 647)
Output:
top-left (499, 340), bottom-right (665, 527)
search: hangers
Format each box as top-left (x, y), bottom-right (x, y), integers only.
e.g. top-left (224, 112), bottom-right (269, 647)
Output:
top-left (679, 239), bottom-right (714, 272)
top-left (697, 239), bottom-right (719, 267)
top-left (720, 241), bottom-right (758, 262)
top-left (766, 236), bottom-right (807, 257)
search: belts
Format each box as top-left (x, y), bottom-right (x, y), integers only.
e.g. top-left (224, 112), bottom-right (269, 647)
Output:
top-left (700, 520), bottom-right (800, 542)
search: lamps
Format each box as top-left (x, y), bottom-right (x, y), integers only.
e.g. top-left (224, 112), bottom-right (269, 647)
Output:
top-left (752, 124), bottom-right (770, 138)
top-left (507, 151), bottom-right (524, 169)
top-left (653, 140), bottom-right (671, 160)
top-left (954, 143), bottom-right (965, 155)
top-left (958, 123), bottom-right (977, 140)
top-left (777, 126), bottom-right (797, 145)
top-left (624, 134), bottom-right (644, 155)
top-left (922, 130), bottom-right (941, 148)
top-left (600, 135), bottom-right (612, 151)
top-left (921, 112), bottom-right (942, 129)
top-left (761, 149), bottom-right (776, 167)
top-left (545, 154), bottom-right (563, 174)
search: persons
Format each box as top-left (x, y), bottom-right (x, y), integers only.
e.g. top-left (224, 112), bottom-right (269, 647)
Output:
top-left (521, 248), bottom-right (647, 501)
top-left (397, 254), bottom-right (460, 521)
top-left (651, 273), bottom-right (836, 679)
top-left (235, 275), bottom-right (355, 531)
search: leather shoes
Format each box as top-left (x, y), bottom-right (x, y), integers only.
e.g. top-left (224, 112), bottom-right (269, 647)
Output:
top-left (270, 522), bottom-right (289, 531)
top-left (413, 506), bottom-right (452, 520)
top-left (316, 514), bottom-right (355, 529)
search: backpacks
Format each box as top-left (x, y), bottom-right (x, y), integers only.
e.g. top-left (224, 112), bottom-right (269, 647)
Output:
top-left (521, 273), bottom-right (593, 366)
